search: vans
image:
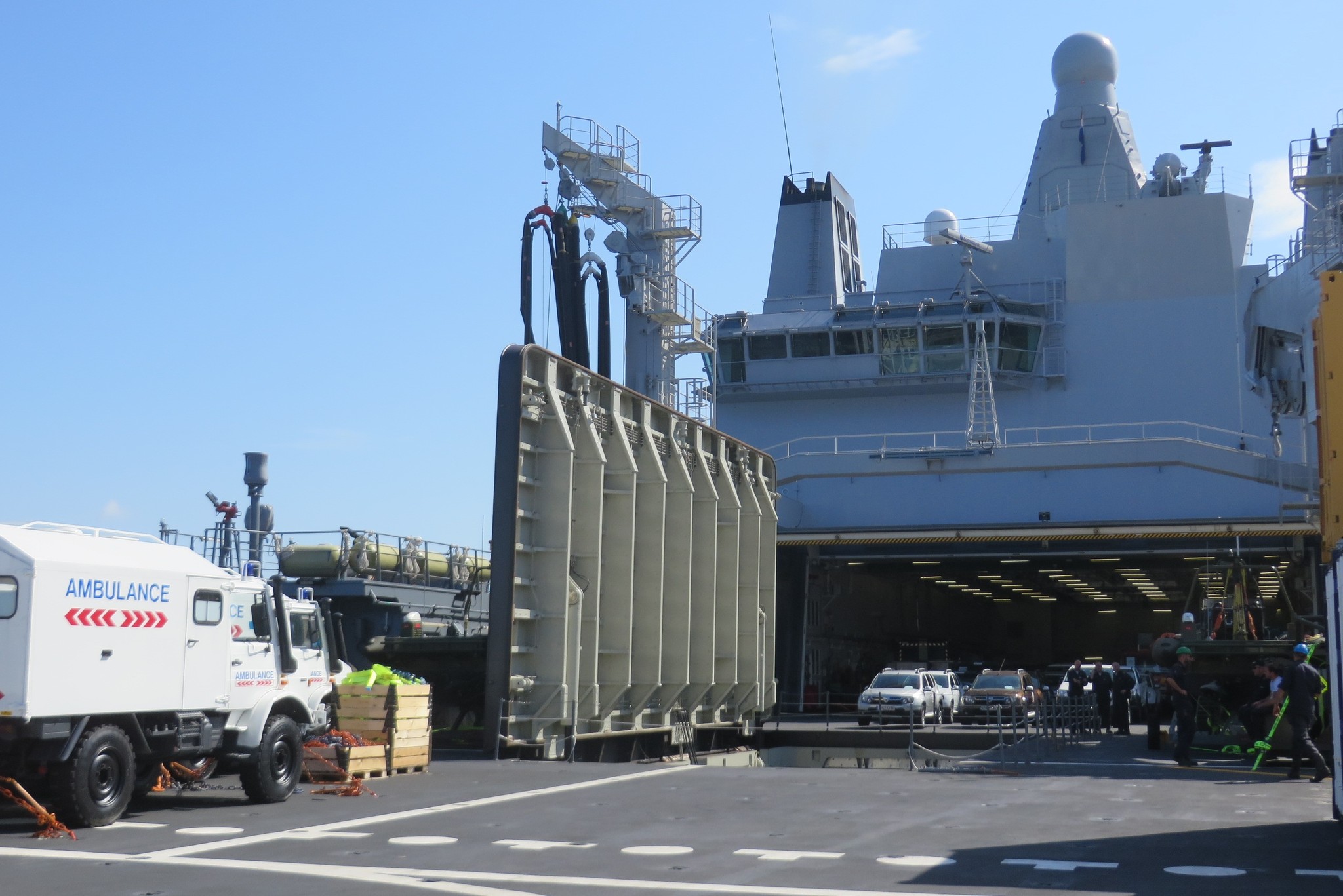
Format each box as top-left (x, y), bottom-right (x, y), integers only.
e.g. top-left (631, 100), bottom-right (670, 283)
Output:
top-left (1029, 664), bottom-right (1173, 728)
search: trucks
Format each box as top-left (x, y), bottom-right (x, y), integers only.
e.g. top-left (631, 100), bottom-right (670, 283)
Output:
top-left (0, 521), bottom-right (356, 830)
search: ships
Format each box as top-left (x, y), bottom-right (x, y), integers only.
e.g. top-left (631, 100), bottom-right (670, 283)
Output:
top-left (690, 12), bottom-right (1328, 722)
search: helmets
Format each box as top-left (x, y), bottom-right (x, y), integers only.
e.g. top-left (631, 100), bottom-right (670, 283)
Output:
top-left (1175, 647), bottom-right (1190, 653)
top-left (1182, 612), bottom-right (1194, 622)
top-left (1295, 643), bottom-right (1309, 654)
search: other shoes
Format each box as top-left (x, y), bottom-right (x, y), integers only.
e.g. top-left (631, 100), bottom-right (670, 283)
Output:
top-left (1287, 772), bottom-right (1300, 780)
top-left (1093, 728), bottom-right (1100, 734)
top-left (1310, 764), bottom-right (1330, 782)
top-left (1114, 729), bottom-right (1130, 735)
top-left (1179, 758), bottom-right (1198, 765)
top-left (1080, 729), bottom-right (1088, 733)
top-left (1070, 728), bottom-right (1075, 733)
top-left (1106, 728), bottom-right (1113, 733)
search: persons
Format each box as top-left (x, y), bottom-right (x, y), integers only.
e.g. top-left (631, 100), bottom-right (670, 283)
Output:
top-left (1166, 646), bottom-right (1198, 765)
top-left (1067, 660), bottom-right (1088, 734)
top-left (1237, 657), bottom-right (1283, 752)
top-left (1087, 661), bottom-right (1136, 735)
top-left (1272, 643), bottom-right (1330, 783)
top-left (1304, 631), bottom-right (1327, 642)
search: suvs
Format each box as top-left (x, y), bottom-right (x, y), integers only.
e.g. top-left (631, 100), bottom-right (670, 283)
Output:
top-left (857, 667), bottom-right (943, 729)
top-left (901, 668), bottom-right (966, 725)
top-left (958, 668), bottom-right (1044, 727)
